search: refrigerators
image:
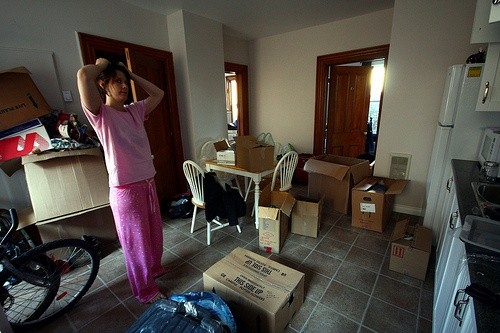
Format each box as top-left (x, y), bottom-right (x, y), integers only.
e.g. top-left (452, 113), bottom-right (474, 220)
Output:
top-left (422, 63), bottom-right (500, 228)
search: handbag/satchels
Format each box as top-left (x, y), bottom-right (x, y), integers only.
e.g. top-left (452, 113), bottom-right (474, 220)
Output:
top-left (257, 133), bottom-right (274, 145)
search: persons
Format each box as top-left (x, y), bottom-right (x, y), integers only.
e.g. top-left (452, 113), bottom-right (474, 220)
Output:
top-left (77, 58), bottom-right (171, 304)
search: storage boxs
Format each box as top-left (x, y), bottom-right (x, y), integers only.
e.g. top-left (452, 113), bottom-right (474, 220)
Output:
top-left (211, 140), bottom-right (235, 165)
top-left (350, 176), bottom-right (411, 233)
top-left (232, 136), bottom-right (275, 175)
top-left (256, 189), bottom-right (297, 254)
top-left (292, 193), bottom-right (329, 238)
top-left (0, 64), bottom-right (120, 260)
top-left (304, 152), bottom-right (370, 215)
top-left (203, 246), bottom-right (305, 333)
top-left (388, 217), bottom-right (435, 279)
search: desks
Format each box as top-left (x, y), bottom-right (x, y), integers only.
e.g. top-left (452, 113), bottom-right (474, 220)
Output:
top-left (204, 158), bottom-right (286, 228)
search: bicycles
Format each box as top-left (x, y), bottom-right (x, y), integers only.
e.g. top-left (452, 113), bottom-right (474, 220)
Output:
top-left (1, 207), bottom-right (100, 333)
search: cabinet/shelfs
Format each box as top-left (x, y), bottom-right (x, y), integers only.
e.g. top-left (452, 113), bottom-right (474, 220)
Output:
top-left (432, 167), bottom-right (477, 333)
top-left (471, 0), bottom-right (500, 112)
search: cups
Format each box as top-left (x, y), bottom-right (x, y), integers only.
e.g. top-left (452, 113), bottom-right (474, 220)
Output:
top-left (477, 161), bottom-right (500, 183)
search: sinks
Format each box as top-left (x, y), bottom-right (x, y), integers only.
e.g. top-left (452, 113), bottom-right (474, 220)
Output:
top-left (478, 203), bottom-right (500, 222)
top-left (471, 181), bottom-right (500, 208)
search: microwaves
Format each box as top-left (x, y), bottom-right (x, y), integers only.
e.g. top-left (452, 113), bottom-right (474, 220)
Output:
top-left (478, 128), bottom-right (500, 177)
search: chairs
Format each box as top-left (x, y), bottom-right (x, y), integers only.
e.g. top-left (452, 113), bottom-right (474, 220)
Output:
top-left (251, 150), bottom-right (298, 219)
top-left (182, 160), bottom-right (242, 244)
top-left (244, 143), bottom-right (283, 201)
top-left (200, 140), bottom-right (243, 197)
